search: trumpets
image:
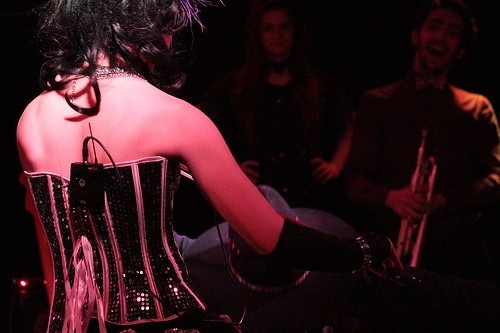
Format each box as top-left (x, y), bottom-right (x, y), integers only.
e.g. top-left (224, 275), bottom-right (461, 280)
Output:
top-left (397, 129), bottom-right (438, 269)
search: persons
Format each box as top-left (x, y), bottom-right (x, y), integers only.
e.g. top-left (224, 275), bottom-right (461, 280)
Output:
top-left (15, 0), bottom-right (395, 333)
top-left (229, 8), bottom-right (356, 209)
top-left (342, 0), bottom-right (500, 223)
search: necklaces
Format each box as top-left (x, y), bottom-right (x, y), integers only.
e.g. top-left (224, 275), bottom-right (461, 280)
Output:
top-left (58, 67), bottom-right (145, 102)
